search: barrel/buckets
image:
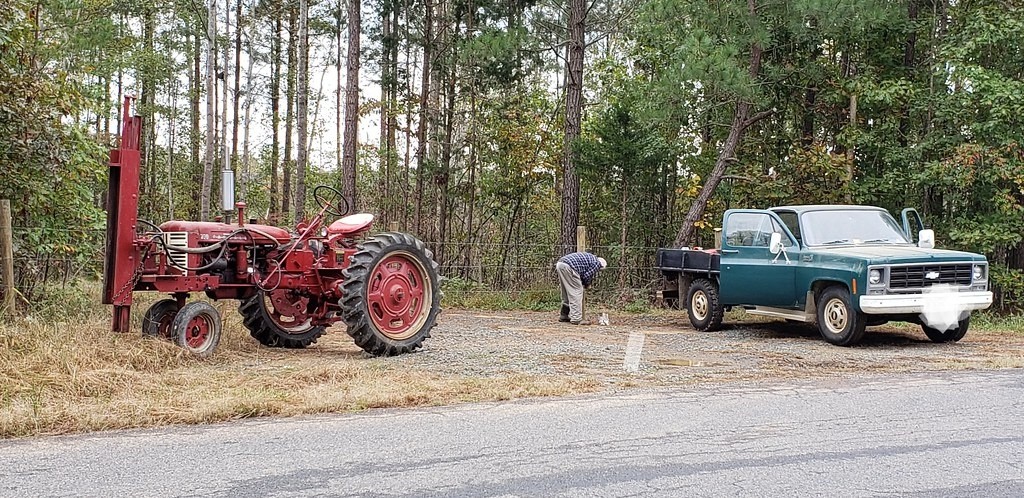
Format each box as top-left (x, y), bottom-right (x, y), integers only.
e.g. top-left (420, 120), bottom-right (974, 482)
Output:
top-left (918, 229), bottom-right (935, 249)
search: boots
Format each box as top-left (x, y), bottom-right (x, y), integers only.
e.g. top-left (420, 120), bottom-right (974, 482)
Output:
top-left (571, 320), bottom-right (582, 325)
top-left (559, 304), bottom-right (571, 322)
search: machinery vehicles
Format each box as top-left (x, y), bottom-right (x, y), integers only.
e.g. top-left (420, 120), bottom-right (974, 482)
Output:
top-left (103, 93), bottom-right (444, 358)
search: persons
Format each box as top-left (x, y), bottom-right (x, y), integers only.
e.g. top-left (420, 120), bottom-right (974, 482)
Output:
top-left (556, 252), bottom-right (607, 325)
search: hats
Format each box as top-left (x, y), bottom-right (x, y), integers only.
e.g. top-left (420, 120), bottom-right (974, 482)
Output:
top-left (598, 258), bottom-right (607, 270)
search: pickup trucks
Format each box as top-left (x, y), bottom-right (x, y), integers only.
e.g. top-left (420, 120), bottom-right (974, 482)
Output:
top-left (655, 205), bottom-right (994, 347)
